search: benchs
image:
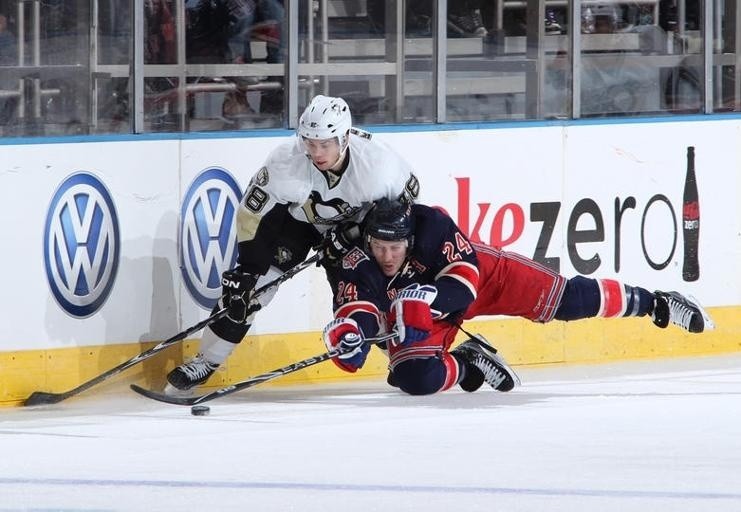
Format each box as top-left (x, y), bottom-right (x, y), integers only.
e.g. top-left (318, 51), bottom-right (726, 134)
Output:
top-left (1, 0), bottom-right (739, 123)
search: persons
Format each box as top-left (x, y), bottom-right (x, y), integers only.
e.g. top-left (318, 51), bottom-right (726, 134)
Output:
top-left (166, 93), bottom-right (419, 394)
top-left (323, 201), bottom-right (706, 396)
top-left (1, 2), bottom-right (702, 125)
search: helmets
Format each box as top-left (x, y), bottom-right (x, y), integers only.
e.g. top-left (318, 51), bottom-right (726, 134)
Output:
top-left (361, 197), bottom-right (416, 253)
top-left (295, 93), bottom-right (353, 155)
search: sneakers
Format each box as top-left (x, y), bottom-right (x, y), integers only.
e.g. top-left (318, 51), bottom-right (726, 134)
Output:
top-left (222, 94), bottom-right (255, 116)
top-left (456, 336), bottom-right (514, 392)
top-left (336, 12), bottom-right (488, 39)
top-left (517, 10), bottom-right (563, 35)
top-left (166, 353), bottom-right (219, 392)
top-left (653, 290), bottom-right (704, 335)
top-left (259, 95), bottom-right (284, 115)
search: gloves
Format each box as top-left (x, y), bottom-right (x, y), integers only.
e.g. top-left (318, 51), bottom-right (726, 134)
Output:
top-left (321, 302), bottom-right (381, 375)
top-left (314, 224), bottom-right (352, 271)
top-left (220, 267), bottom-right (262, 326)
top-left (385, 288), bottom-right (437, 349)
